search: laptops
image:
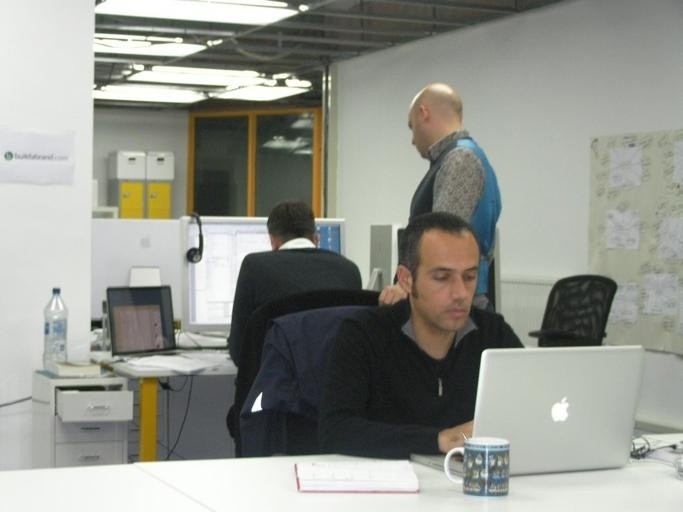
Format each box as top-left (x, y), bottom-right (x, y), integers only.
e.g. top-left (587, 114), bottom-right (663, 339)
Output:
top-left (106, 285), bottom-right (187, 357)
top-left (411, 344), bottom-right (644, 472)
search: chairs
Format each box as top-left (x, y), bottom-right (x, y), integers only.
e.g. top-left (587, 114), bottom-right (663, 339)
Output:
top-left (527, 269), bottom-right (618, 349)
top-left (234, 287), bottom-right (379, 434)
top-left (233, 302), bottom-right (408, 462)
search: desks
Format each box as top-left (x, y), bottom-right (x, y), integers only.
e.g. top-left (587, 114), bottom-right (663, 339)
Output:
top-left (0, 429), bottom-right (682, 512)
top-left (93, 337), bottom-right (247, 463)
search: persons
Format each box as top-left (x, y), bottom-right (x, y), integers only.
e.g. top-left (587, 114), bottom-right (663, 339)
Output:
top-left (221, 199), bottom-right (362, 438)
top-left (378, 82), bottom-right (502, 315)
top-left (315, 209), bottom-right (527, 461)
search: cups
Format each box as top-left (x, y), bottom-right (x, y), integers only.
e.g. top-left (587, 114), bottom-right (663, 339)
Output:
top-left (443, 437), bottom-right (512, 495)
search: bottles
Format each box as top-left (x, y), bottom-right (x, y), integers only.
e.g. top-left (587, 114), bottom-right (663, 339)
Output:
top-left (100, 299), bottom-right (110, 352)
top-left (41, 287), bottom-right (69, 365)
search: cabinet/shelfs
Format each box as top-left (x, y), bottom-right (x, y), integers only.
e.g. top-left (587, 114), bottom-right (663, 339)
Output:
top-left (27, 367), bottom-right (136, 471)
top-left (109, 177), bottom-right (174, 222)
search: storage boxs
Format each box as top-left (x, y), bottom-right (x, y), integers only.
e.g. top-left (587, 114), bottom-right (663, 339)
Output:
top-left (139, 150), bottom-right (174, 181)
top-left (106, 148), bottom-right (145, 181)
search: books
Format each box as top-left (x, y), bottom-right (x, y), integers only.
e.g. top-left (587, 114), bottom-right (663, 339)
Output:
top-left (293, 456), bottom-right (420, 495)
top-left (133, 351), bottom-right (224, 376)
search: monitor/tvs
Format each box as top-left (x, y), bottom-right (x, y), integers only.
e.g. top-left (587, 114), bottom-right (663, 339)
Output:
top-left (180, 216), bottom-right (347, 333)
top-left (367, 223), bottom-right (399, 291)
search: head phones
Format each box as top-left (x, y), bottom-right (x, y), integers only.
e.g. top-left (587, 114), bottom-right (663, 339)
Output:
top-left (186, 211), bottom-right (203, 262)
